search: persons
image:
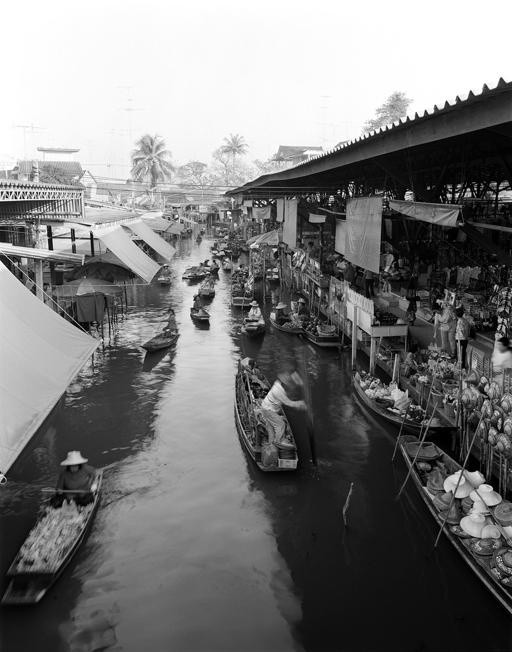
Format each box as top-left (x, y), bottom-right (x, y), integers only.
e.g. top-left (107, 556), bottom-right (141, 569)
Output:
top-left (306, 234), bottom-right (512, 396)
top-left (261, 372), bottom-right (306, 443)
top-left (196, 228), bottom-right (238, 255)
top-left (162, 263), bottom-right (171, 278)
top-left (247, 301), bottom-right (261, 319)
top-left (163, 309), bottom-right (176, 338)
top-left (274, 298), bottom-right (310, 324)
top-left (51, 450), bottom-right (95, 508)
top-left (192, 258), bottom-right (249, 314)
top-left (241, 357), bottom-right (256, 373)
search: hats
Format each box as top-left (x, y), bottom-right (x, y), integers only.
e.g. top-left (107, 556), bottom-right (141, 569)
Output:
top-left (460, 369), bottom-right (512, 454)
top-left (169, 308), bottom-right (174, 313)
top-left (427, 470), bottom-right (512, 588)
top-left (276, 372), bottom-right (291, 386)
top-left (249, 300), bottom-right (259, 307)
top-left (60, 450), bottom-right (89, 467)
top-left (297, 298), bottom-right (306, 304)
top-left (193, 293), bottom-right (200, 301)
top-left (275, 302), bottom-right (288, 309)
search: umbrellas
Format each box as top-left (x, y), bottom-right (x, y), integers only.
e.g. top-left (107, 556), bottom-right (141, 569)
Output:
top-left (51, 276), bottom-right (123, 298)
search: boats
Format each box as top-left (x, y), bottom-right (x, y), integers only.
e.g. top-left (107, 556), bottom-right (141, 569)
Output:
top-left (396, 433), bottom-right (512, 614)
top-left (157, 276), bottom-right (171, 285)
top-left (182, 266), bottom-right (219, 280)
top-left (198, 280), bottom-right (215, 297)
top-left (351, 370), bottom-right (460, 436)
top-left (230, 264), bottom-right (254, 308)
top-left (222, 256), bottom-right (233, 270)
top-left (233, 358), bottom-right (299, 474)
top-left (3, 469), bottom-right (105, 605)
top-left (251, 251), bottom-right (280, 282)
top-left (241, 312), bottom-right (265, 337)
top-left (290, 300), bottom-right (343, 347)
top-left (211, 227), bottom-right (249, 259)
top-left (191, 307), bottom-right (210, 321)
top-left (141, 332), bottom-right (180, 350)
top-left (269, 312), bottom-right (305, 334)
